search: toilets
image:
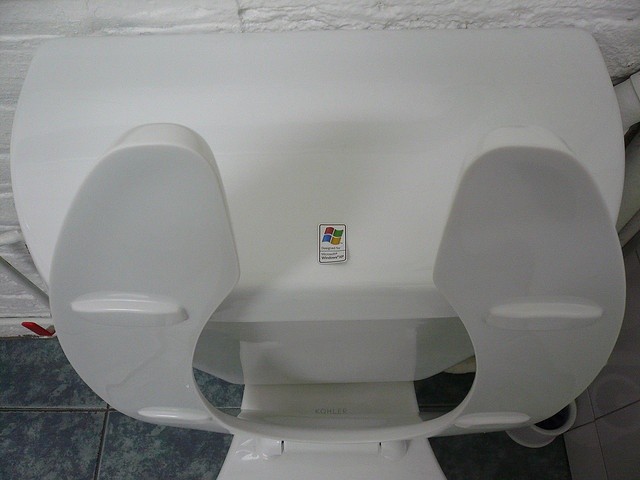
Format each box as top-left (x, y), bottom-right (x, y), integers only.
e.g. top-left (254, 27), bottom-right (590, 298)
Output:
top-left (9, 27), bottom-right (629, 480)
